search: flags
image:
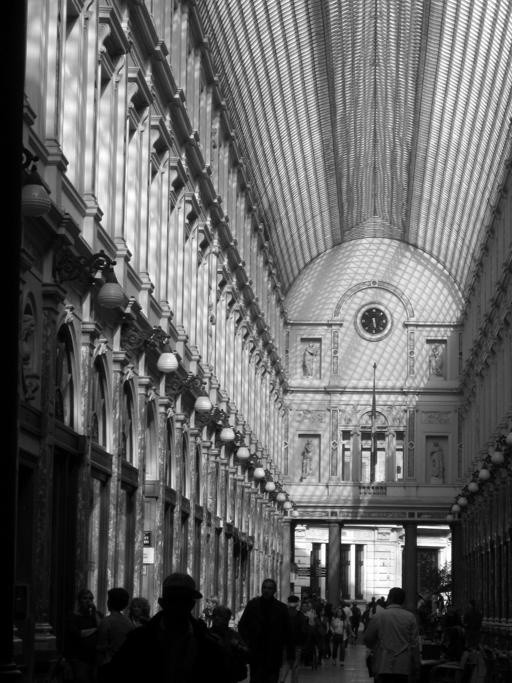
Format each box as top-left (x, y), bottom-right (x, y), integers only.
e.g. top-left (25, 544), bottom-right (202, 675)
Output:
top-left (369, 366), bottom-right (379, 488)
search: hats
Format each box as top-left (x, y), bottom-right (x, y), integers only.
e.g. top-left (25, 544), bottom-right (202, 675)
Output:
top-left (159, 572), bottom-right (203, 601)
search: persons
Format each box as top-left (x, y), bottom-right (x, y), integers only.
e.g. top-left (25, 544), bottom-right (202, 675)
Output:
top-left (428, 343), bottom-right (444, 378)
top-left (52, 572), bottom-right (361, 683)
top-left (428, 438), bottom-right (445, 485)
top-left (361, 587), bottom-right (482, 682)
top-left (301, 437), bottom-right (316, 481)
top-left (302, 341), bottom-right (319, 378)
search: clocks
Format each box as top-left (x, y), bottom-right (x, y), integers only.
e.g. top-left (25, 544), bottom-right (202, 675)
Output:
top-left (353, 300), bottom-right (395, 343)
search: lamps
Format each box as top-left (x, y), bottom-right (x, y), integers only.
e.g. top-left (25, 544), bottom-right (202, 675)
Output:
top-left (51, 247), bottom-right (126, 311)
top-left (122, 323), bottom-right (298, 522)
top-left (445, 431), bottom-right (510, 530)
top-left (19, 144), bottom-right (52, 221)
